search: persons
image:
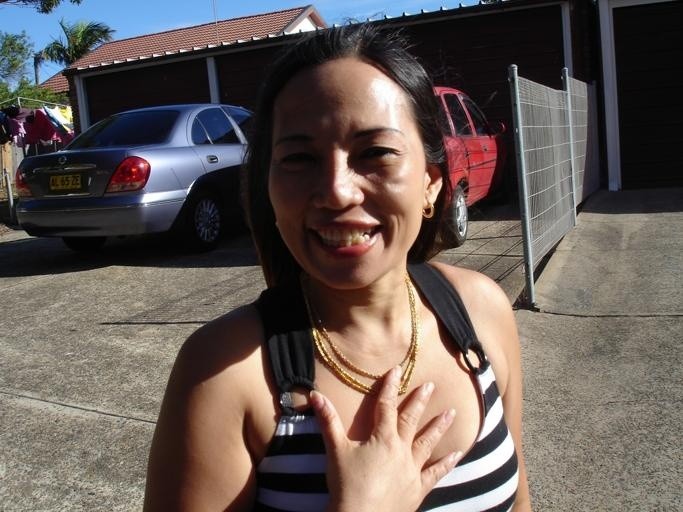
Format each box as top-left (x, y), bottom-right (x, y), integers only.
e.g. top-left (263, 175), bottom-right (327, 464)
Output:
top-left (139, 20), bottom-right (535, 512)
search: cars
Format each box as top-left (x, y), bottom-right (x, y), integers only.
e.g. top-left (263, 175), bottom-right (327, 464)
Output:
top-left (15, 103), bottom-right (256, 253)
top-left (432, 87), bottom-right (513, 248)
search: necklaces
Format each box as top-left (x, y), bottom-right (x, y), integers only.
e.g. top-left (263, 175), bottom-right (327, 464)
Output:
top-left (299, 270), bottom-right (419, 401)
